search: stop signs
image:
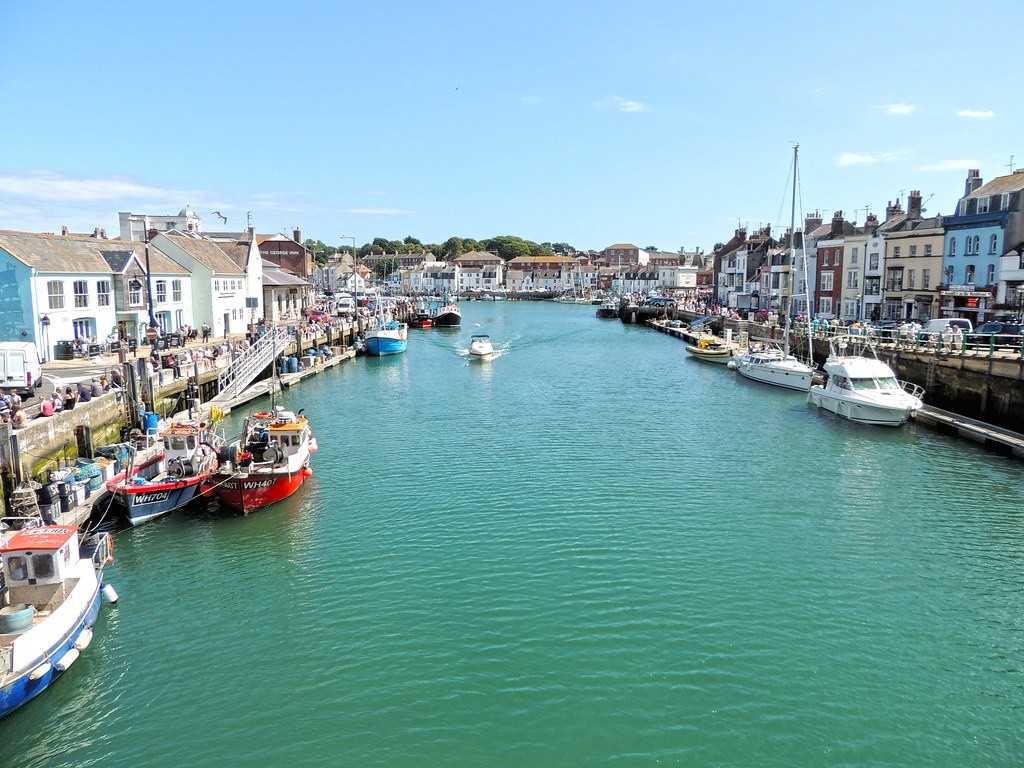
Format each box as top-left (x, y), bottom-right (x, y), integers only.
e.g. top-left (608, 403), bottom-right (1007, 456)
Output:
top-left (42, 317), bottom-right (50, 325)
top-left (146, 328), bottom-right (157, 340)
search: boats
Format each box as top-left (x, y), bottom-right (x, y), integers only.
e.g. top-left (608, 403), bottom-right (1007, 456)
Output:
top-left (469, 334), bottom-right (493, 355)
top-left (807, 335), bottom-right (926, 426)
top-left (685, 142), bottom-right (819, 391)
top-left (364, 291), bottom-right (407, 355)
top-left (553, 255), bottom-right (621, 317)
top-left (0, 323), bottom-right (318, 717)
top-left (410, 302), bottom-right (461, 328)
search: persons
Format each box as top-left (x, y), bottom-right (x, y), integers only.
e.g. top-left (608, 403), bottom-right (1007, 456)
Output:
top-left (236, 309), bottom-right (364, 357)
top-left (0, 388), bottom-right (28, 429)
top-left (150, 343), bottom-right (233, 386)
top-left (34, 371), bottom-right (121, 419)
top-left (179, 322), bottom-right (209, 347)
top-left (685, 293), bottom-right (806, 334)
top-left (813, 315), bottom-right (963, 353)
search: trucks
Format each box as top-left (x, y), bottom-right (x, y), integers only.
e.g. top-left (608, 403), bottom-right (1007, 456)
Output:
top-left (338, 298), bottom-right (368, 317)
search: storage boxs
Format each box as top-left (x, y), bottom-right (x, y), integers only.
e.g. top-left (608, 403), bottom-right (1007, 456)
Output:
top-left (34, 479), bottom-right (91, 522)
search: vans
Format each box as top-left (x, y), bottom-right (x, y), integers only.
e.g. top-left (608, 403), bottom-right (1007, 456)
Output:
top-left (0, 341), bottom-right (43, 397)
top-left (921, 318), bottom-right (973, 350)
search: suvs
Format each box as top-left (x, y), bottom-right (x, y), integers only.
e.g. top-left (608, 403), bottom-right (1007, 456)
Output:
top-left (967, 315), bottom-right (1024, 350)
top-left (845, 318), bottom-right (924, 343)
top-left (779, 314), bottom-right (802, 327)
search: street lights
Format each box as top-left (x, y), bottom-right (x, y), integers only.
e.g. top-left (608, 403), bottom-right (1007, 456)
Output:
top-left (128, 217), bottom-right (159, 362)
top-left (340, 236), bottom-right (358, 321)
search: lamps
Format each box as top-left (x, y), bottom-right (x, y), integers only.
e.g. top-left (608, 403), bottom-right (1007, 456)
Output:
top-left (39, 313), bottom-right (51, 325)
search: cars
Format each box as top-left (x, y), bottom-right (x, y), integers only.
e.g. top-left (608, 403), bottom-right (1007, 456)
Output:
top-left (638, 297), bottom-right (678, 308)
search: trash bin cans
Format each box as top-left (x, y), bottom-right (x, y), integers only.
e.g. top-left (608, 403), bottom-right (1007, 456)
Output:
top-left (57, 340), bottom-right (74, 360)
